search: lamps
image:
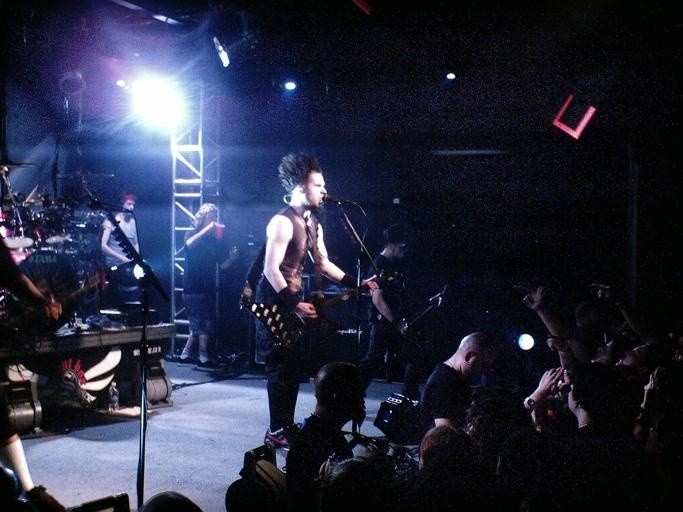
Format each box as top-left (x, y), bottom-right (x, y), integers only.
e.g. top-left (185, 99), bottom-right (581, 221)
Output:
top-left (213, 11), bottom-right (264, 71)
top-left (54, 38), bottom-right (85, 95)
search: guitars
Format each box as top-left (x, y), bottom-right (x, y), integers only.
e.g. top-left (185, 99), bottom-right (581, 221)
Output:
top-left (0, 249), bottom-right (107, 342)
top-left (392, 285), bottom-right (447, 342)
top-left (234, 271), bottom-right (405, 352)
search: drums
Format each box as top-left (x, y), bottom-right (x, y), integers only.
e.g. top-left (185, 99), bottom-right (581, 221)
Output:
top-left (20, 248), bottom-right (89, 317)
top-left (3, 203), bottom-right (34, 248)
top-left (43, 208), bottom-right (74, 243)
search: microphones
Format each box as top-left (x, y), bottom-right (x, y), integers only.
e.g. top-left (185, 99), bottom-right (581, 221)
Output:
top-left (321, 192), bottom-right (351, 208)
top-left (3, 158), bottom-right (38, 171)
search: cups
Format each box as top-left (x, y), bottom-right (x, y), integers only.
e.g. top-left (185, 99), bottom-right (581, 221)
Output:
top-left (216, 224), bottom-right (225, 239)
top-left (109, 381), bottom-right (120, 409)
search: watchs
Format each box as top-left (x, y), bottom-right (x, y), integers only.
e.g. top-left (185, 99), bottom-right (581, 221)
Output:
top-left (525, 397), bottom-right (538, 408)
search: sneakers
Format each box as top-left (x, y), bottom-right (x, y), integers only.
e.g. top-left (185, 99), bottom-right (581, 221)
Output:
top-left (264, 427), bottom-right (293, 449)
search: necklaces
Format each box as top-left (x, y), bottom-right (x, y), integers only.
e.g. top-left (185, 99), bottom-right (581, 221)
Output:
top-left (580, 424), bottom-right (587, 429)
top-left (311, 412), bottom-right (329, 423)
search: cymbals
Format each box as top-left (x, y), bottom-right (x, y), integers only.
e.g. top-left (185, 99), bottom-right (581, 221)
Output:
top-left (54, 171), bottom-right (115, 179)
top-left (0, 162), bottom-right (35, 169)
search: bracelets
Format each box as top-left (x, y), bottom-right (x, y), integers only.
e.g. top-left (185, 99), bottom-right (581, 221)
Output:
top-left (275, 286), bottom-right (301, 309)
top-left (635, 408), bottom-right (649, 428)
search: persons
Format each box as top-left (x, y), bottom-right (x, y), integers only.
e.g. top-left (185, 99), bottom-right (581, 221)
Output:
top-left (0, 232), bottom-right (69, 512)
top-left (282, 276), bottom-right (681, 512)
top-left (238, 149), bottom-right (383, 454)
top-left (98, 193), bottom-right (145, 311)
top-left (178, 202), bottom-right (238, 370)
top-left (357, 227), bottom-right (429, 405)
